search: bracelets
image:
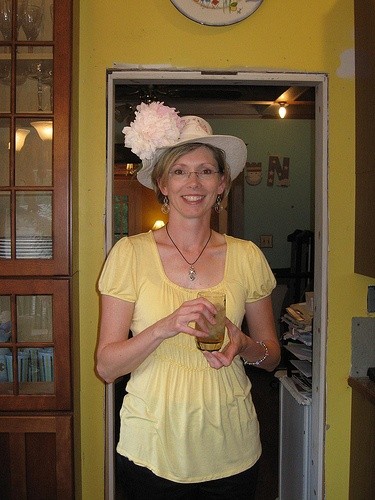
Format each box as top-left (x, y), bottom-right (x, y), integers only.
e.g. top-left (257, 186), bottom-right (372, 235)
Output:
top-left (243, 341), bottom-right (269, 366)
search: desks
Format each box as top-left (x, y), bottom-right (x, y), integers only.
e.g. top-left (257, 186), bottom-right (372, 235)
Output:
top-left (346, 377), bottom-right (375, 500)
top-left (274, 371), bottom-right (308, 500)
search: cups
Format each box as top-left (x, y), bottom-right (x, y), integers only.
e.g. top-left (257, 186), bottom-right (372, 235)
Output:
top-left (0, 348), bottom-right (54, 383)
top-left (194, 291), bottom-right (226, 352)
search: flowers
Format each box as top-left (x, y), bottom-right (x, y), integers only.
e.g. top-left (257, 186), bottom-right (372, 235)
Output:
top-left (119, 102), bottom-right (181, 158)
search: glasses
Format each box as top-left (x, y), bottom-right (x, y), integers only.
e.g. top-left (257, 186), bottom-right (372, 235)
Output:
top-left (167, 167), bottom-right (222, 180)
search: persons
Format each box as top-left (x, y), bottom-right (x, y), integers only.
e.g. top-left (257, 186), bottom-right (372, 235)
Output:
top-left (95, 103), bottom-right (282, 500)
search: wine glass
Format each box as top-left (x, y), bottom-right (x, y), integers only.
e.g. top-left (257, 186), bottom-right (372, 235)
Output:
top-left (25, 62), bottom-right (53, 112)
top-left (0, 4), bottom-right (42, 54)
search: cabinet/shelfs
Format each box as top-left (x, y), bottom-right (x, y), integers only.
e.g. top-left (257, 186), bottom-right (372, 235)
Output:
top-left (111, 164), bottom-right (244, 242)
top-left (352, 0), bottom-right (375, 277)
top-left (0, 0), bottom-right (73, 500)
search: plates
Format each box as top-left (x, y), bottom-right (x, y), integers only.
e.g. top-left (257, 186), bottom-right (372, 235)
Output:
top-left (0, 235), bottom-right (53, 259)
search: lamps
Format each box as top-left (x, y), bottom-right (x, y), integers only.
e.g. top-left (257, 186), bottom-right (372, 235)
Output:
top-left (30, 121), bottom-right (53, 140)
top-left (7, 129), bottom-right (30, 151)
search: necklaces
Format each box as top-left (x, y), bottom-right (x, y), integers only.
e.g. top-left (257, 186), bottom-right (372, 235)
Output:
top-left (166, 221), bottom-right (212, 281)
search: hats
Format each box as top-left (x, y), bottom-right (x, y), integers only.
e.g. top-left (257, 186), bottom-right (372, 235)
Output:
top-left (122, 100), bottom-right (248, 191)
